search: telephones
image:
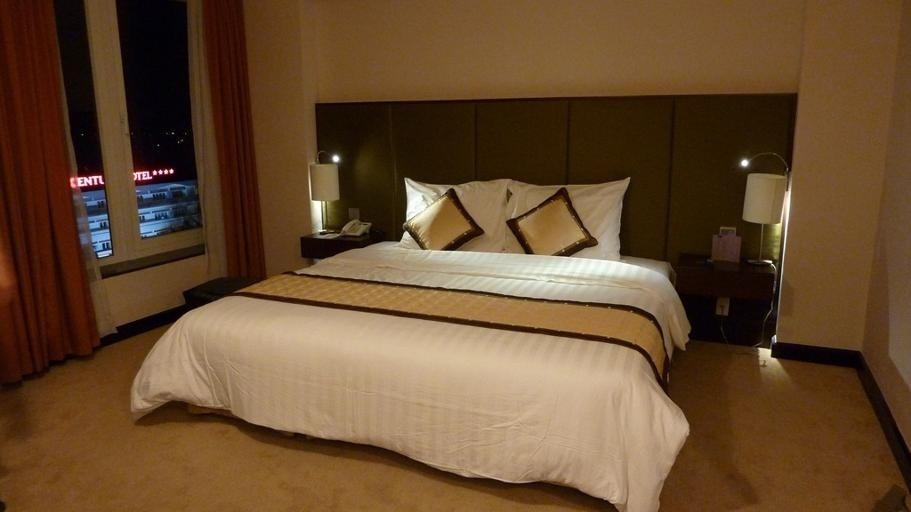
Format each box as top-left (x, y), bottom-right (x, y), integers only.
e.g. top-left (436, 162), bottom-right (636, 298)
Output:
top-left (340, 219), bottom-right (372, 237)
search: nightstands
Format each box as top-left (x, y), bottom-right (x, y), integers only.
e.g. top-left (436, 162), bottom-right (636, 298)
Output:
top-left (674, 253), bottom-right (775, 347)
top-left (301, 226), bottom-right (383, 259)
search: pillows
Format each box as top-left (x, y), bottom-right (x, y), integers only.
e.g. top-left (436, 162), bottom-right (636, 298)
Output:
top-left (395, 176), bottom-right (633, 263)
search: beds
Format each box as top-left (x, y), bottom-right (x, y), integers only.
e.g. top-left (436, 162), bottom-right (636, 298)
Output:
top-left (179, 238), bottom-right (678, 500)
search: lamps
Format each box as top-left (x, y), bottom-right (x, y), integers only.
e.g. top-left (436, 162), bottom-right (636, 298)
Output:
top-left (742, 172), bottom-right (789, 265)
top-left (309, 163), bottom-right (342, 230)
